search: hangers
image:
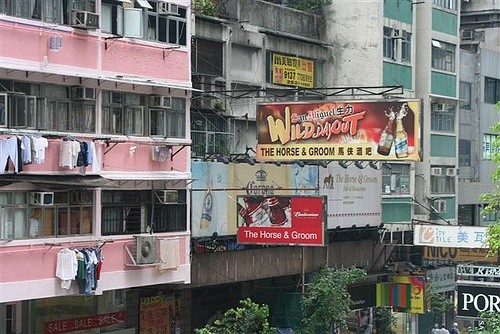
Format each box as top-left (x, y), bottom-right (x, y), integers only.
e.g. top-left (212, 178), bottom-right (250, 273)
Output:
top-left (60, 133), bottom-right (96, 146)
top-left (0, 130), bottom-right (48, 142)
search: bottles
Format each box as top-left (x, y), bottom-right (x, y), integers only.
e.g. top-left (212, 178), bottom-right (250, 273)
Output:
top-left (378, 117), bottom-right (395, 155)
top-left (266, 197), bottom-right (288, 226)
top-left (237, 203), bottom-right (253, 227)
top-left (242, 196), bottom-right (270, 227)
top-left (395, 120), bottom-right (409, 159)
top-left (200, 183), bottom-right (214, 231)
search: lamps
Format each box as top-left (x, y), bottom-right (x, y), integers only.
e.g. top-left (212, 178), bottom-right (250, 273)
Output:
top-left (203, 153), bottom-right (392, 170)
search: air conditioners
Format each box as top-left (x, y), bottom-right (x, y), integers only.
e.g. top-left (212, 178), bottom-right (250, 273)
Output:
top-left (159, 190), bottom-right (178, 204)
top-left (150, 96), bottom-right (172, 109)
top-left (463, 31), bottom-right (474, 39)
top-left (133, 235), bottom-right (156, 264)
top-left (434, 200), bottom-right (447, 214)
top-left (30, 192), bottom-right (54, 207)
top-left (72, 87), bottom-right (95, 100)
top-left (433, 103), bottom-right (449, 113)
top-left (71, 11), bottom-right (100, 29)
top-left (430, 168), bottom-right (442, 176)
top-left (159, 1), bottom-right (178, 15)
top-left (445, 168), bottom-right (456, 176)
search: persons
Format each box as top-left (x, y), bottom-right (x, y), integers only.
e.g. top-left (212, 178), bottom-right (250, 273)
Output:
top-left (438, 324), bottom-right (450, 334)
top-left (432, 324), bottom-right (439, 334)
top-left (450, 323), bottom-right (460, 334)
